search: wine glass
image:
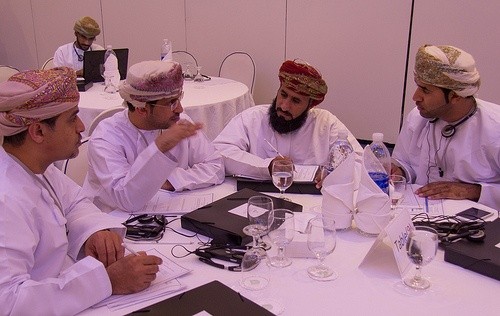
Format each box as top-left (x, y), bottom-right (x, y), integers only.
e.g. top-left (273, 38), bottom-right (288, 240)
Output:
top-left (267, 209), bottom-right (295, 268)
top-left (193, 66), bottom-right (205, 89)
top-left (183, 62), bottom-right (194, 85)
top-left (306, 217), bottom-right (337, 278)
top-left (403, 226), bottom-right (438, 289)
top-left (272, 159), bottom-right (294, 203)
top-left (390, 175), bottom-right (406, 215)
top-left (241, 224), bottom-right (271, 290)
top-left (246, 196), bottom-right (274, 251)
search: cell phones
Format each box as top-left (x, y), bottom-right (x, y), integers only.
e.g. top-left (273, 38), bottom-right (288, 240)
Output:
top-left (455, 206), bottom-right (494, 222)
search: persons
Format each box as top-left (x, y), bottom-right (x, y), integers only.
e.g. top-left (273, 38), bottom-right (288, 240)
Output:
top-left (82, 59), bottom-right (225, 215)
top-left (0, 67), bottom-right (163, 316)
top-left (390, 45), bottom-right (500, 212)
top-left (212, 59), bottom-right (363, 190)
top-left (54, 16), bottom-right (105, 77)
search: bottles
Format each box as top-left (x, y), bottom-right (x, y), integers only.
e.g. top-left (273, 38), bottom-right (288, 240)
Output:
top-left (104, 45), bottom-right (116, 65)
top-left (322, 129), bottom-right (353, 186)
top-left (364, 133), bottom-right (391, 195)
top-left (160, 39), bottom-right (170, 62)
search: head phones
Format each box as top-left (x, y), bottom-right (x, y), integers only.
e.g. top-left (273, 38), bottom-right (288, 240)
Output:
top-left (195, 244), bottom-right (266, 271)
top-left (73, 41), bottom-right (93, 61)
top-left (432, 217), bottom-right (486, 242)
top-left (121, 213), bottom-right (168, 238)
top-left (428, 94), bottom-right (477, 138)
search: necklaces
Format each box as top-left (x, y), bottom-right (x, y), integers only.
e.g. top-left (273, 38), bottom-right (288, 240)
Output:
top-left (73, 41), bottom-right (92, 60)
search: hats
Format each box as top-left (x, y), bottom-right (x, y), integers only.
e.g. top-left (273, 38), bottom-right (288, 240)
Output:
top-left (118, 61), bottom-right (185, 108)
top-left (277, 57), bottom-right (328, 110)
top-left (414, 44), bottom-right (481, 98)
top-left (0, 66), bottom-right (80, 136)
top-left (73, 16), bottom-right (101, 39)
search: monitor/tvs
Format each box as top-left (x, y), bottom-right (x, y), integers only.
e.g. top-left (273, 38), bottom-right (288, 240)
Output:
top-left (83, 48), bottom-right (129, 83)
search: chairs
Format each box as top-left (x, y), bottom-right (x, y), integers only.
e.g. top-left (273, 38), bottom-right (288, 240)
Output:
top-left (0, 56), bottom-right (53, 82)
top-left (62, 107), bottom-right (125, 185)
top-left (171, 51), bottom-right (202, 75)
top-left (220, 50), bottom-right (256, 100)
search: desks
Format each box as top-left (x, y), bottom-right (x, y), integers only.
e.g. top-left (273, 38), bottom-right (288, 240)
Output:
top-left (73, 177), bottom-right (500, 315)
top-left (55, 72), bottom-right (255, 169)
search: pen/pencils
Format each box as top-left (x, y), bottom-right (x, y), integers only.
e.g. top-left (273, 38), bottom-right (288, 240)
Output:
top-left (265, 138), bottom-right (297, 174)
top-left (226, 197), bottom-right (249, 201)
top-left (120, 242), bottom-right (140, 256)
top-left (424, 197), bottom-right (428, 213)
